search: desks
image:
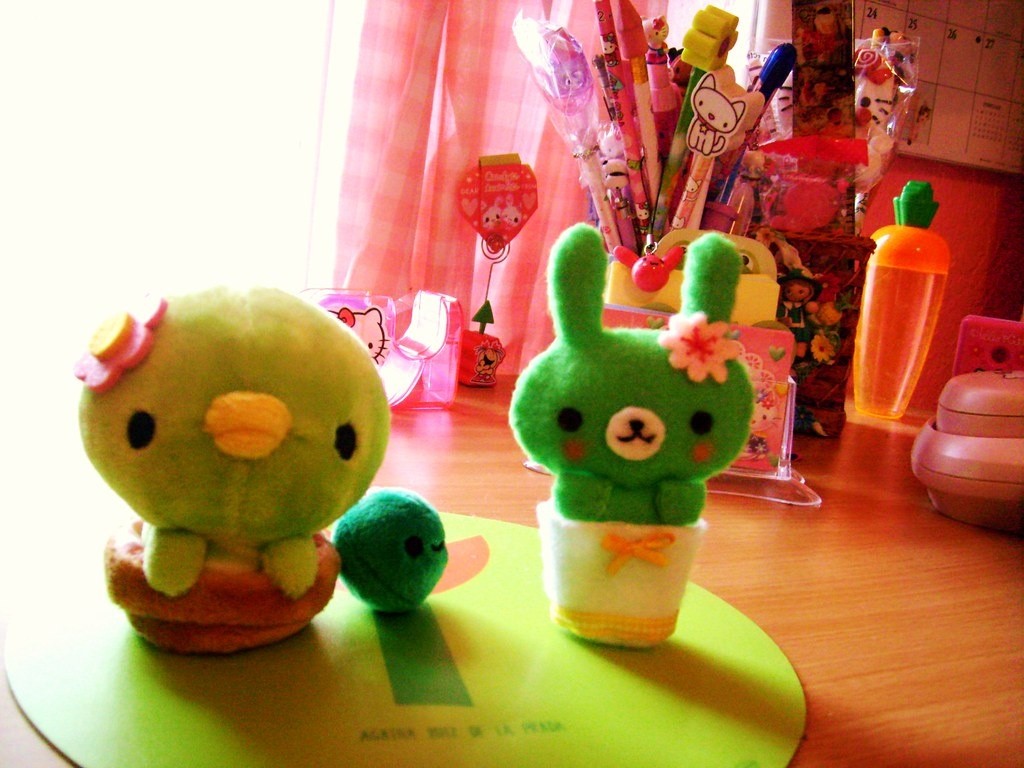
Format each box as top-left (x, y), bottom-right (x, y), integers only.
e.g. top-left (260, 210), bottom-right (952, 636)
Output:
top-left (0, 376), bottom-right (1024, 768)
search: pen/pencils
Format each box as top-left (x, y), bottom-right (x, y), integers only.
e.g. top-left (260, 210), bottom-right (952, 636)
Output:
top-left (507, 0), bottom-right (795, 262)
